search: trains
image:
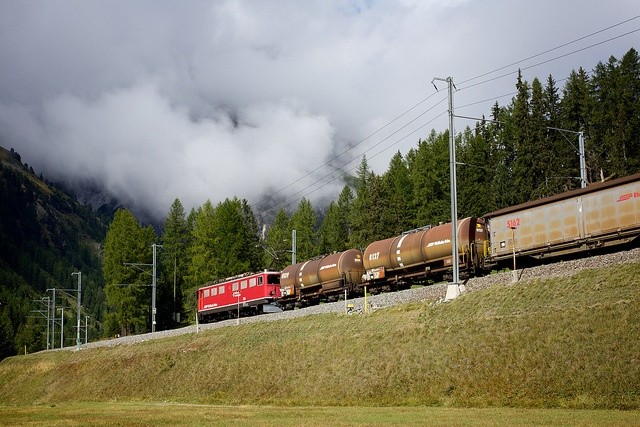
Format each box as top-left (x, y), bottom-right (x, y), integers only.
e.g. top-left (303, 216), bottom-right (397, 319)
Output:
top-left (196, 172), bottom-right (640, 360)
